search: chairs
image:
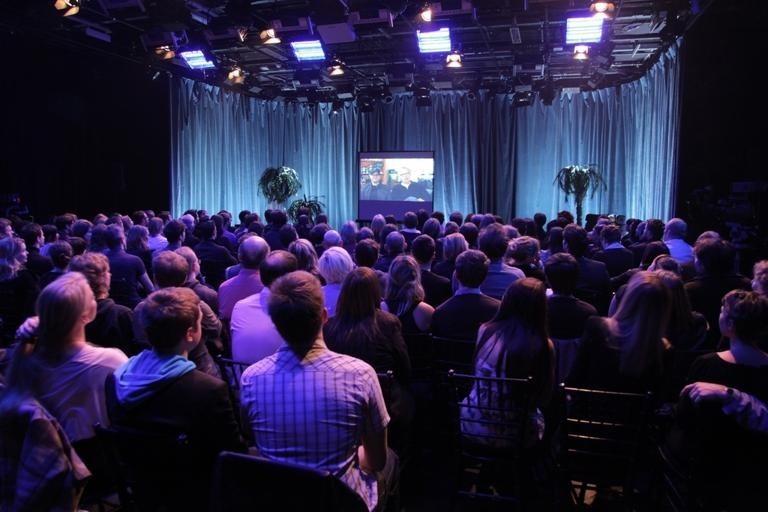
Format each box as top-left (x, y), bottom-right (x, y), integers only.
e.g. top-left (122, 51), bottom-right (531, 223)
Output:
top-left (92, 275), bottom-right (669, 512)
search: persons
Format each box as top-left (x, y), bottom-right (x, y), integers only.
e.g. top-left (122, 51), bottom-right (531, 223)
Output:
top-left (390, 167), bottom-right (431, 202)
top-left (360, 166), bottom-right (392, 201)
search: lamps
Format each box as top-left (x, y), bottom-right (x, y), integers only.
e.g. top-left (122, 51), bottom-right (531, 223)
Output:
top-left (163, 10), bottom-right (617, 117)
